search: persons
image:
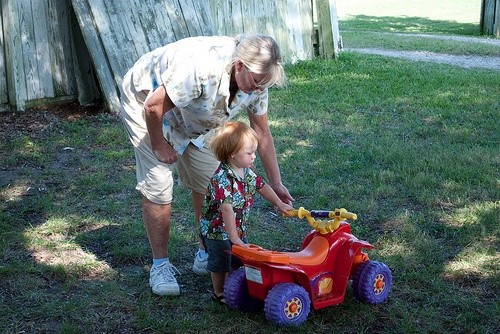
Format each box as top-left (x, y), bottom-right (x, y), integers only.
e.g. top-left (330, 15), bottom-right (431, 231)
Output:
top-left (198, 121), bottom-right (294, 305)
top-left (118, 35), bottom-right (295, 295)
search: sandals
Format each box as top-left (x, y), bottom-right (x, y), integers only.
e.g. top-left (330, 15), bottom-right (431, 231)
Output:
top-left (210, 292), bottom-right (229, 309)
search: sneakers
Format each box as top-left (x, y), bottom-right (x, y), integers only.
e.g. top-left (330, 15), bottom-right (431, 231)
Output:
top-left (192, 250), bottom-right (211, 275)
top-left (149, 261), bottom-right (182, 295)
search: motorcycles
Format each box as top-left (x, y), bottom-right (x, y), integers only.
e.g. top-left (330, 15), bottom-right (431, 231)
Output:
top-left (222, 206), bottom-right (393, 331)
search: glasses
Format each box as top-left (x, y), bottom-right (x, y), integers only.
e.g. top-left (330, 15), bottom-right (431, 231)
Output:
top-left (248, 68), bottom-right (263, 88)
